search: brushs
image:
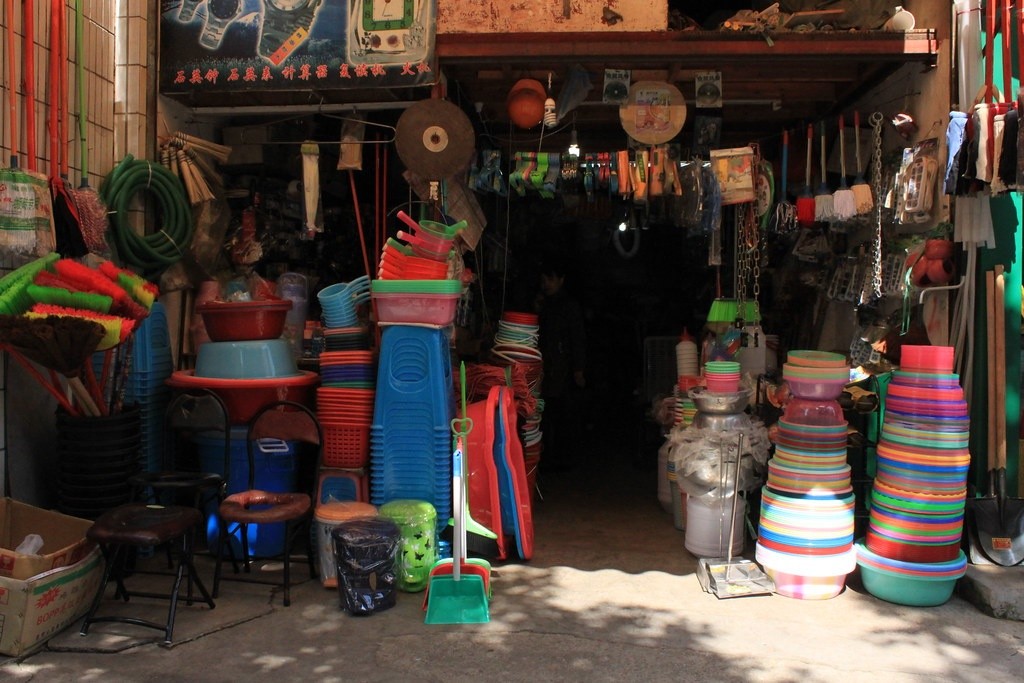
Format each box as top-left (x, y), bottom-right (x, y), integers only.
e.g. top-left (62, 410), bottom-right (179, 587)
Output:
top-left (775, 109), bottom-right (874, 229)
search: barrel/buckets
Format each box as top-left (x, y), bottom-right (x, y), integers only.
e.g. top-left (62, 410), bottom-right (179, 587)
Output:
top-left (486, 312), bottom-right (542, 499)
top-left (201, 432), bottom-right (293, 557)
top-left (54, 400), bottom-right (143, 579)
top-left (686, 481), bottom-right (746, 557)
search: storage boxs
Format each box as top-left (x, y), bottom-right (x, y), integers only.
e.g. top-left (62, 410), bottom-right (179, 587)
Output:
top-left (0, 496), bottom-right (106, 658)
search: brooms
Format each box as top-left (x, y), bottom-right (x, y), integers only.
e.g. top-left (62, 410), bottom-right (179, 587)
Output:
top-left (44, 0), bottom-right (91, 259)
top-left (439, 361), bottom-right (503, 560)
top-left (705, 206), bottom-right (762, 323)
top-left (0, 252), bottom-right (160, 417)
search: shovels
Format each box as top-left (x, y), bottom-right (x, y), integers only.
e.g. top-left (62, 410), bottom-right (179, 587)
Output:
top-left (964, 263), bottom-right (1024, 569)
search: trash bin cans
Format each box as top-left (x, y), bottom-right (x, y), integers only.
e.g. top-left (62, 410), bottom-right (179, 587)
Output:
top-left (330, 516), bottom-right (401, 617)
top-left (313, 501), bottom-right (379, 588)
top-left (378, 499), bottom-right (438, 593)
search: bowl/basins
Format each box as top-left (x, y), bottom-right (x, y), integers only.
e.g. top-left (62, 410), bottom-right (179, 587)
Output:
top-left (856, 345), bottom-right (969, 607)
top-left (690, 411), bottom-right (755, 432)
top-left (687, 386), bottom-right (753, 415)
top-left (165, 295), bottom-right (316, 420)
top-left (754, 351), bottom-right (855, 596)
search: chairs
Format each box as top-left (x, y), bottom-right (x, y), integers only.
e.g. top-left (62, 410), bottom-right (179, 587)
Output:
top-left (80, 385), bottom-right (325, 645)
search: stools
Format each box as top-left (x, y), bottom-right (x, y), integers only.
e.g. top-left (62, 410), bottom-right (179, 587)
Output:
top-left (368, 325), bottom-right (455, 560)
top-left (84, 302), bottom-right (174, 557)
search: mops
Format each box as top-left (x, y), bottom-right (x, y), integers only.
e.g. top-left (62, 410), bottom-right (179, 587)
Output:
top-left (2, 0), bottom-right (56, 260)
top-left (73, 1), bottom-right (111, 257)
top-left (943, 2), bottom-right (1024, 275)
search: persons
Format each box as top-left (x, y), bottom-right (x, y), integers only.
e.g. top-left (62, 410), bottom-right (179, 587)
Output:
top-left (530, 263), bottom-right (589, 493)
